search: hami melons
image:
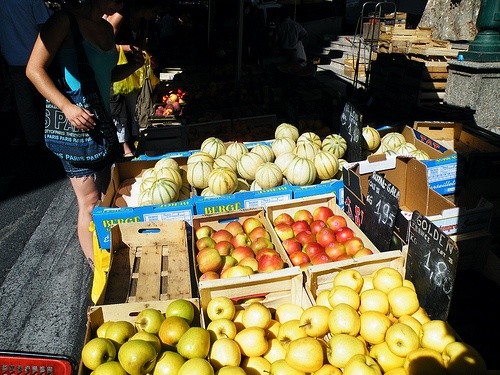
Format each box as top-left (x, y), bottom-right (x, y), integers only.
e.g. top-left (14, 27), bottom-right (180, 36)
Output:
top-left (114, 157), bottom-right (191, 208)
top-left (187, 123), bottom-right (347, 197)
top-left (362, 125), bottom-right (431, 161)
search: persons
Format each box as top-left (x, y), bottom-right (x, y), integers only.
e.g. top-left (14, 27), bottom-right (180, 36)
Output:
top-left (0, 0), bottom-right (191, 158)
top-left (245, 5), bottom-right (266, 44)
top-left (271, 4), bottom-right (310, 60)
top-left (24, 0), bottom-right (125, 274)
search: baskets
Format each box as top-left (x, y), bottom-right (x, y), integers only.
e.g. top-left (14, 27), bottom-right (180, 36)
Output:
top-left (0, 351), bottom-right (74, 375)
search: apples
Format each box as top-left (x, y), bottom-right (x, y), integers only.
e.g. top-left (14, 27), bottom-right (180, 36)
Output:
top-left (154, 86), bottom-right (187, 115)
top-left (82, 299), bottom-right (214, 375)
top-left (196, 218), bottom-right (284, 281)
top-left (207, 296), bottom-right (343, 375)
top-left (274, 207), bottom-right (373, 268)
top-left (299, 267), bottom-right (488, 375)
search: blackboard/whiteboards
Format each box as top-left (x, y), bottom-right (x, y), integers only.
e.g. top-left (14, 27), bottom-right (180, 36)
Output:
top-left (340, 101), bottom-right (364, 162)
top-left (405, 209), bottom-right (460, 323)
top-left (362, 171), bottom-right (401, 252)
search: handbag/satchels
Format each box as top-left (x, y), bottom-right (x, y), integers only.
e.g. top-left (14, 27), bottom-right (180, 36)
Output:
top-left (43, 10), bottom-right (117, 162)
top-left (134, 77), bottom-right (158, 129)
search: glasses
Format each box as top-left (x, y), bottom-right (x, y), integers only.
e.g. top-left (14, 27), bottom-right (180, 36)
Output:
top-left (113, 0), bottom-right (127, 5)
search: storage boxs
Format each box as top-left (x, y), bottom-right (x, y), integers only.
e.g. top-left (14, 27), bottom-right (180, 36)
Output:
top-left (0, 68), bottom-right (500, 375)
